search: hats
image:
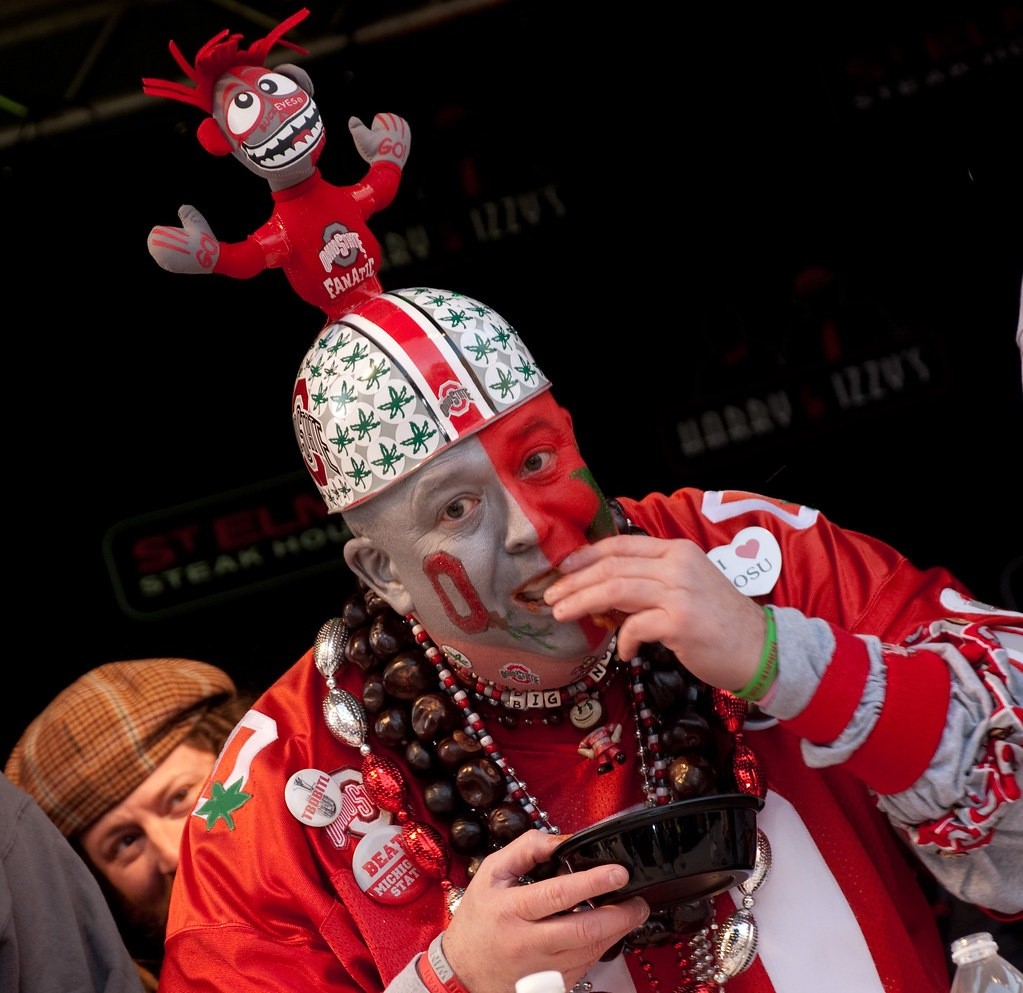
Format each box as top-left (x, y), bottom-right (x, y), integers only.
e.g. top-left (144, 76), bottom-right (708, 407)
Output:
top-left (5, 658), bottom-right (238, 843)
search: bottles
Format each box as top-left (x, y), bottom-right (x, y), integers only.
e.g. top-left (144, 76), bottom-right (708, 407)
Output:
top-left (948, 933), bottom-right (1023, 993)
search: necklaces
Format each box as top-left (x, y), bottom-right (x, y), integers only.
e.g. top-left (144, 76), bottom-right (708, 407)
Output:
top-left (312, 581), bottom-right (772, 993)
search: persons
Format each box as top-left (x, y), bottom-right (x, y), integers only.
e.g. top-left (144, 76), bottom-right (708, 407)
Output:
top-left (5, 655), bottom-right (248, 993)
top-left (157, 283), bottom-right (1022, 993)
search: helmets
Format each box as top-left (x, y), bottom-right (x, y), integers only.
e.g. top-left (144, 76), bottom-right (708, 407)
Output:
top-left (290, 284), bottom-right (554, 517)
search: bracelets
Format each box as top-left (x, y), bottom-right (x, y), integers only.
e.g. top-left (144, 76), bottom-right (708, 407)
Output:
top-left (732, 608), bottom-right (780, 700)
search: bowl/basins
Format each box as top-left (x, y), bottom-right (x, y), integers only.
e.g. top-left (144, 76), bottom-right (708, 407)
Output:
top-left (524, 792), bottom-right (767, 924)
